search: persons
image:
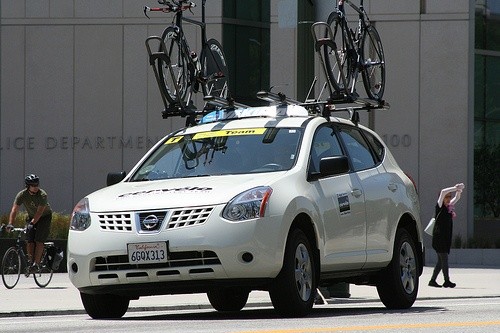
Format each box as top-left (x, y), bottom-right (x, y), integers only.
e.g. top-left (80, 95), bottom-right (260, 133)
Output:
top-left (427, 182), bottom-right (464, 288)
top-left (193, 150), bottom-right (216, 174)
top-left (5, 173), bottom-right (52, 278)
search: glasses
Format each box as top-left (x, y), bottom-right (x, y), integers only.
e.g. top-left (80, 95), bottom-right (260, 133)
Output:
top-left (30, 184), bottom-right (38, 187)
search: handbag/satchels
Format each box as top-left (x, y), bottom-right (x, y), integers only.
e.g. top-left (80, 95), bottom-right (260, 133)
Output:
top-left (424, 217), bottom-right (435, 236)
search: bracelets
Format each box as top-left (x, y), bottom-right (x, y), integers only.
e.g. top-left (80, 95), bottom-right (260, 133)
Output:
top-left (29, 221), bottom-right (33, 225)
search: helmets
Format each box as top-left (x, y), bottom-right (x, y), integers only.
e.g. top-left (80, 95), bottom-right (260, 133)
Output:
top-left (25, 174), bottom-right (39, 185)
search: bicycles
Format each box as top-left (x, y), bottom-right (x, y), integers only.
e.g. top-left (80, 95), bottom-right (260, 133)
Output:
top-left (144, 0), bottom-right (232, 127)
top-left (0, 222), bottom-right (57, 290)
top-left (311, 0), bottom-right (387, 103)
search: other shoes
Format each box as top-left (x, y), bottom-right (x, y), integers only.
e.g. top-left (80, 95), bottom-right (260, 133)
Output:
top-left (27, 263), bottom-right (39, 273)
top-left (429, 279), bottom-right (442, 287)
top-left (443, 281), bottom-right (456, 288)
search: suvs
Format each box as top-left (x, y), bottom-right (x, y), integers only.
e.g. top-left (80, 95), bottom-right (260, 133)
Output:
top-left (67, 90), bottom-right (426, 320)
